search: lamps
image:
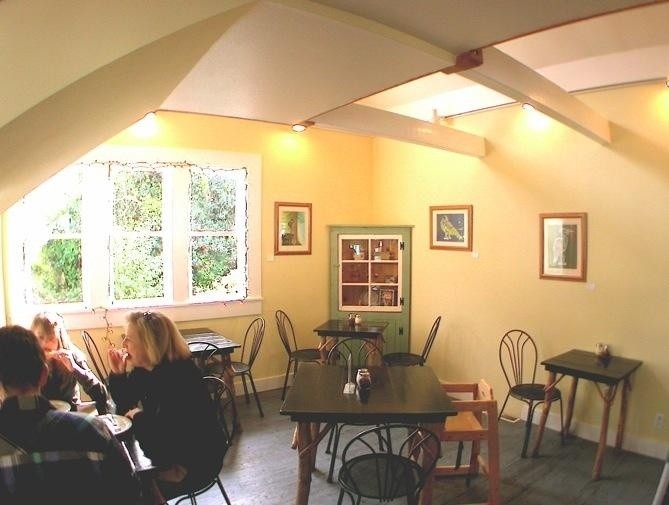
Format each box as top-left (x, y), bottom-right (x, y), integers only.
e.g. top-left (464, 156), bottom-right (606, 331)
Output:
top-left (291, 119), bottom-right (316, 132)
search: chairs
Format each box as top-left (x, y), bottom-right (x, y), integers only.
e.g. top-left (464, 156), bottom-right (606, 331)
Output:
top-left (404, 379), bottom-right (502, 504)
top-left (216, 316), bottom-right (266, 417)
top-left (335, 422), bottom-right (441, 505)
top-left (498, 328), bottom-right (566, 458)
top-left (158, 372), bottom-right (238, 505)
top-left (320, 336), bottom-right (386, 365)
top-left (184, 341), bottom-right (229, 391)
top-left (273, 309), bottom-right (337, 399)
top-left (378, 315), bottom-right (442, 365)
top-left (80, 328), bottom-right (130, 390)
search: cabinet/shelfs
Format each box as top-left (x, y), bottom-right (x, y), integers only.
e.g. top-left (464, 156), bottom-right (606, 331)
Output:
top-left (334, 232), bottom-right (403, 313)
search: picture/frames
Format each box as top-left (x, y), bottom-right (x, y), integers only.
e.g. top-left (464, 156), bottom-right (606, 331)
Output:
top-left (428, 205), bottom-right (473, 252)
top-left (273, 200), bottom-right (313, 256)
top-left (538, 212), bottom-right (587, 282)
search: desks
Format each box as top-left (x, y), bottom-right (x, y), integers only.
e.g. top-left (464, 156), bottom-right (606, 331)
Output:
top-left (279, 361), bottom-right (458, 504)
top-left (177, 326), bottom-right (242, 433)
top-left (313, 318), bottom-right (389, 364)
top-left (532, 346), bottom-right (645, 478)
top-left (73, 400), bottom-right (159, 504)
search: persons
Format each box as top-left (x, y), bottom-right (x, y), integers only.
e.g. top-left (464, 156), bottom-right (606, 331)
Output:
top-left (1, 325), bottom-right (141, 504)
top-left (106, 309), bottom-right (227, 499)
top-left (31, 309), bottom-right (110, 411)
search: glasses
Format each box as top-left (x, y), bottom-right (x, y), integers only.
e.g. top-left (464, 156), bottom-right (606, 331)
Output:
top-left (143, 309), bottom-right (162, 345)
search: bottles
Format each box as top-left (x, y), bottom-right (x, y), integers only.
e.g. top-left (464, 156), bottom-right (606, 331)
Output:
top-left (356, 367), bottom-right (373, 389)
top-left (346, 312), bottom-right (362, 325)
